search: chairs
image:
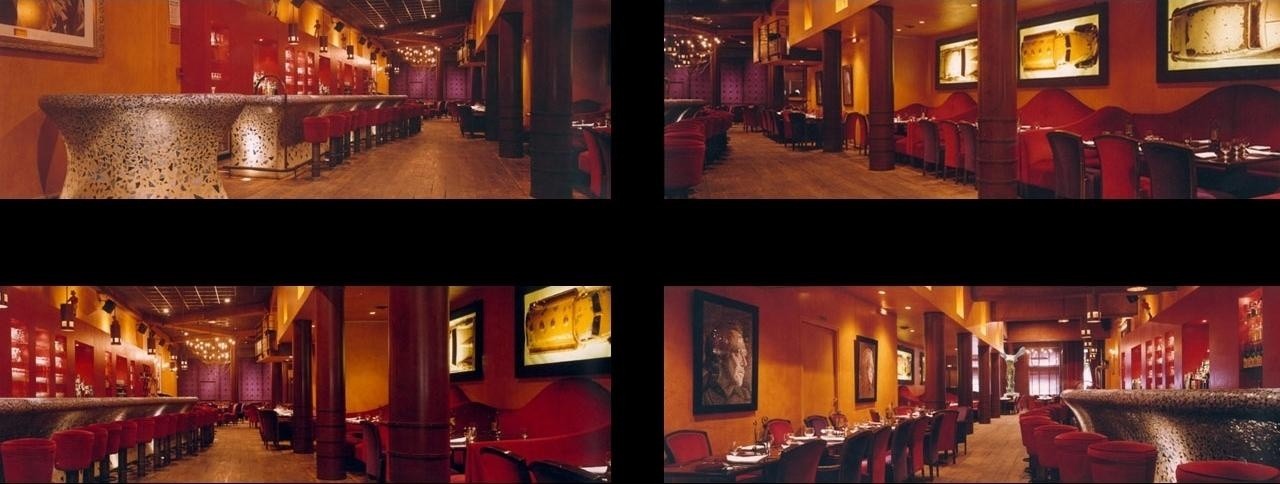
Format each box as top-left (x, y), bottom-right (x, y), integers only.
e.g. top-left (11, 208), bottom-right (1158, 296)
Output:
top-left (1000, 390), bottom-right (1058, 414)
top-left (344, 421), bottom-right (611, 484)
top-left (407, 96), bottom-right (486, 138)
top-left (217, 401), bottom-right (292, 449)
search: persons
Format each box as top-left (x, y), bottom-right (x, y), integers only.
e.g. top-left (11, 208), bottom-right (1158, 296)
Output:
top-left (858, 347), bottom-right (876, 399)
top-left (704, 320), bottom-right (753, 405)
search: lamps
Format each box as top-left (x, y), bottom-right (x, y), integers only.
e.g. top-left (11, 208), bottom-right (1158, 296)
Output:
top-left (288, 0), bottom-right (400, 77)
top-left (0, 285), bottom-right (188, 371)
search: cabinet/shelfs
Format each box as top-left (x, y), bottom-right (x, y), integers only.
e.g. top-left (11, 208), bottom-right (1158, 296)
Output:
top-left (1145, 287), bottom-right (1263, 391)
top-left (252, 47), bottom-right (317, 95)
top-left (10, 317), bottom-right (69, 397)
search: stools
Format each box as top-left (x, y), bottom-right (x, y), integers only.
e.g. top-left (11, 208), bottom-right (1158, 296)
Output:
top-left (304, 102), bottom-right (421, 182)
top-left (1020, 399), bottom-right (1280, 484)
top-left (0, 410), bottom-right (216, 484)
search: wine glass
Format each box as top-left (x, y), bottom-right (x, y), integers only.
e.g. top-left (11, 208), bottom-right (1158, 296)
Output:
top-left (465, 425), bottom-right (478, 444)
top-left (1219, 139), bottom-right (1252, 161)
top-left (781, 403), bottom-right (928, 451)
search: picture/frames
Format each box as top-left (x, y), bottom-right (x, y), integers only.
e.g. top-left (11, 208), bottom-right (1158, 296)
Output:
top-left (856, 336), bottom-right (927, 403)
top-left (693, 289), bottom-right (758, 412)
top-left (815, 64), bottom-right (853, 106)
top-left (0, 0), bottom-right (105, 58)
